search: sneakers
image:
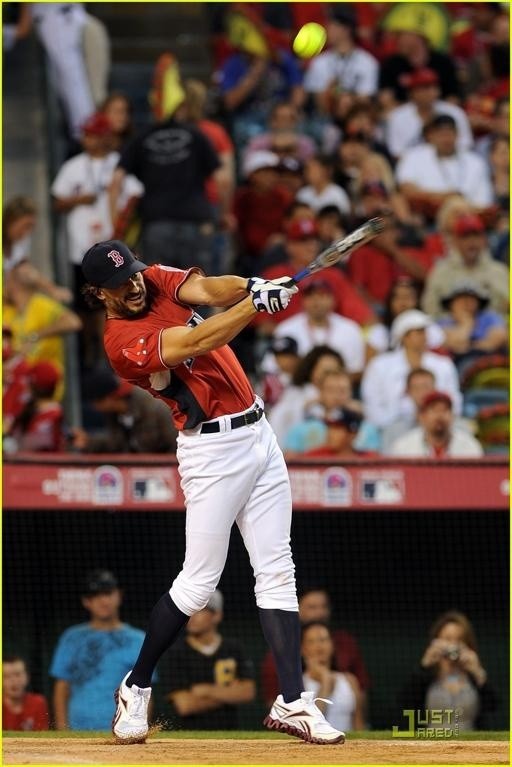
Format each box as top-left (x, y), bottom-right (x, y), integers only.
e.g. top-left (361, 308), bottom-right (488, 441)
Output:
top-left (110, 665), bottom-right (156, 745)
top-left (260, 688), bottom-right (348, 745)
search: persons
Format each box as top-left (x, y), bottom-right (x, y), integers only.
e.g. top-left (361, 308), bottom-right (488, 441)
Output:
top-left (2, 655), bottom-right (53, 729)
top-left (264, 585), bottom-right (370, 728)
top-left (81, 240), bottom-right (347, 743)
top-left (301, 621), bottom-right (367, 728)
top-left (46, 567), bottom-right (159, 730)
top-left (0, 2), bottom-right (510, 465)
top-left (397, 613), bottom-right (501, 729)
top-left (160, 587), bottom-right (258, 730)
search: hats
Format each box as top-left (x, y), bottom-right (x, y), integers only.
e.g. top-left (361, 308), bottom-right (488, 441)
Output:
top-left (439, 275), bottom-right (492, 312)
top-left (398, 64), bottom-right (441, 88)
top-left (79, 237), bottom-right (152, 292)
top-left (286, 216), bottom-right (322, 241)
top-left (236, 146), bottom-right (282, 180)
top-left (301, 273), bottom-right (337, 293)
top-left (78, 109), bottom-right (113, 135)
top-left (453, 213), bottom-right (486, 238)
top-left (28, 358), bottom-right (65, 391)
top-left (206, 587), bottom-right (226, 613)
top-left (418, 390), bottom-right (453, 412)
top-left (389, 307), bottom-right (433, 348)
top-left (75, 565), bottom-right (124, 599)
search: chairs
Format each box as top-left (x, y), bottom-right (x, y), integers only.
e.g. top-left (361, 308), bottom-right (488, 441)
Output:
top-left (461, 354), bottom-right (510, 456)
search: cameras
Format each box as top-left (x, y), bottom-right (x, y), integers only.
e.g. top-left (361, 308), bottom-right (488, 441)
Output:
top-left (440, 643), bottom-right (462, 662)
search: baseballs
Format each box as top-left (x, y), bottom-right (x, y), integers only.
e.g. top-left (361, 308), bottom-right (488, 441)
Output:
top-left (293, 22), bottom-right (327, 59)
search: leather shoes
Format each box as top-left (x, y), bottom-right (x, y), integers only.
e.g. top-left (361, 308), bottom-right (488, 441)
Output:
top-left (199, 401), bottom-right (264, 434)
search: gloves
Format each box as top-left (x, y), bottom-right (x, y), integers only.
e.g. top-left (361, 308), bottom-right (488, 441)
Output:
top-left (246, 274), bottom-right (303, 293)
top-left (252, 275), bottom-right (297, 315)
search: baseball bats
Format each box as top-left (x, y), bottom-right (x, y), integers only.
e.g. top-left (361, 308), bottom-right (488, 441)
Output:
top-left (284, 218), bottom-right (386, 288)
